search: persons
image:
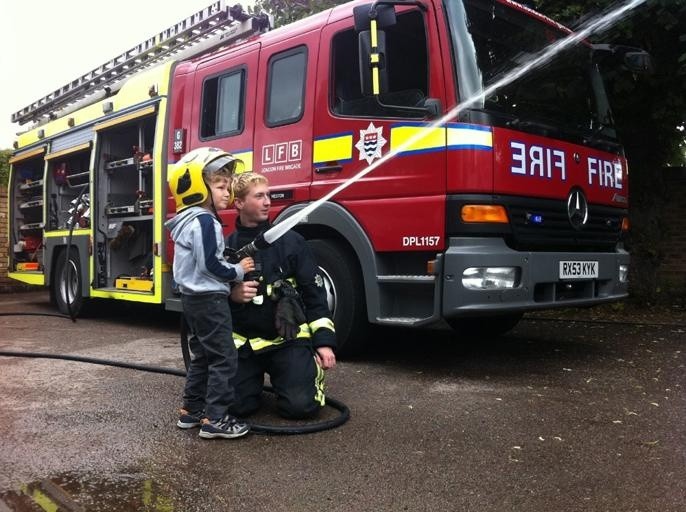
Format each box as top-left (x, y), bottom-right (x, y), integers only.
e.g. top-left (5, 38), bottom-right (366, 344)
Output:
top-left (224, 172), bottom-right (336, 420)
top-left (164, 147), bottom-right (256, 438)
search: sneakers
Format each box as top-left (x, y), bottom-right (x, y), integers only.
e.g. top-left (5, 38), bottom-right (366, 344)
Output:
top-left (198, 414), bottom-right (251, 440)
top-left (177, 408), bottom-right (218, 430)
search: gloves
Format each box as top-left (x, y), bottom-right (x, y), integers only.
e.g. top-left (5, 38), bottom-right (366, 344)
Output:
top-left (274, 285), bottom-right (306, 341)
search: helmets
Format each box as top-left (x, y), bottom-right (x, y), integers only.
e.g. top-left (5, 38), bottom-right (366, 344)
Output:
top-left (168, 146), bottom-right (246, 214)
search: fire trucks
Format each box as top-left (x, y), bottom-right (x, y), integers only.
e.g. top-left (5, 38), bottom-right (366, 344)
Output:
top-left (4, 0), bottom-right (643, 357)
